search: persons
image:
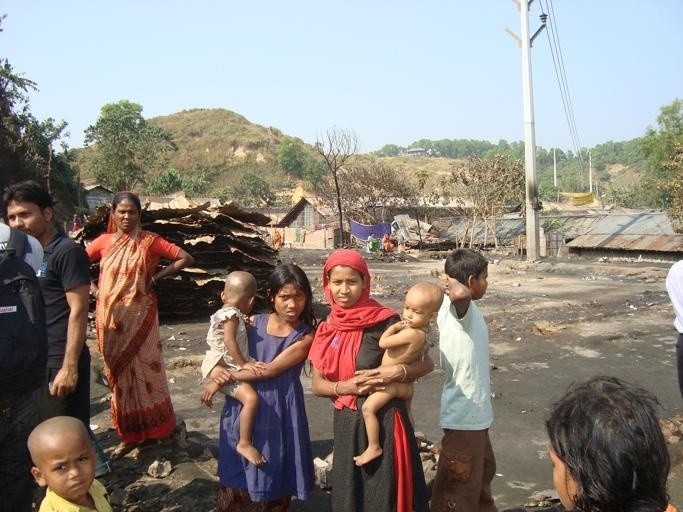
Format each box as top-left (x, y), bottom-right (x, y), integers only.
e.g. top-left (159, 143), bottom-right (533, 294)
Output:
top-left (365, 233), bottom-right (374, 259)
top-left (201, 271), bottom-right (268, 466)
top-left (274, 230), bottom-right (282, 251)
top-left (382, 233), bottom-right (394, 252)
top-left (545, 375), bottom-right (679, 512)
top-left (352, 281), bottom-right (444, 467)
top-left (84, 191), bottom-right (194, 462)
top-left (308, 249), bottom-right (428, 511)
top-left (665, 259), bottom-right (683, 401)
top-left (1, 180), bottom-right (112, 511)
top-left (217, 264), bottom-right (315, 512)
top-left (430, 248), bottom-right (499, 512)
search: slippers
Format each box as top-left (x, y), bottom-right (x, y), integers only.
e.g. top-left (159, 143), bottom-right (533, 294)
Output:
top-left (110, 439), bottom-right (137, 461)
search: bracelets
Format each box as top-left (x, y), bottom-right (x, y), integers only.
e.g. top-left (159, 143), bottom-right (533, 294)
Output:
top-left (400, 364), bottom-right (406, 384)
top-left (333, 381), bottom-right (343, 397)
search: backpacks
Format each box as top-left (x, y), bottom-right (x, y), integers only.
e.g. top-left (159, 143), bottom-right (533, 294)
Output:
top-left (0, 248), bottom-right (50, 395)
